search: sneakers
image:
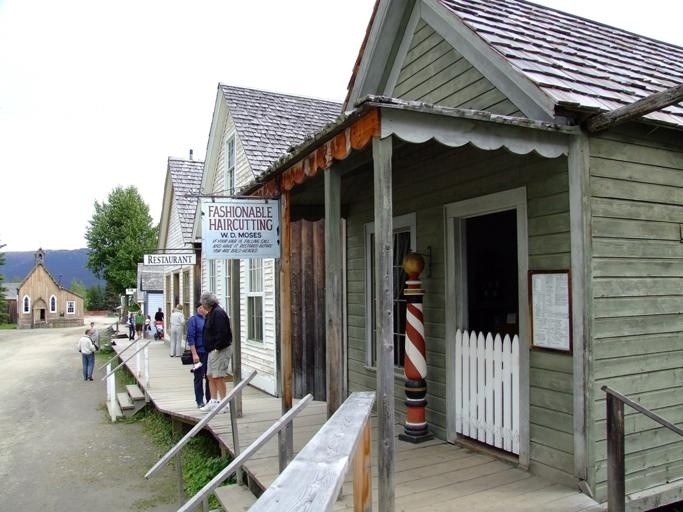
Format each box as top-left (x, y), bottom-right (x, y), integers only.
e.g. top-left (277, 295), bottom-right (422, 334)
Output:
top-left (87, 377), bottom-right (93, 381)
top-left (211, 405), bottom-right (230, 414)
top-left (201, 401), bottom-right (217, 412)
top-left (198, 403), bottom-right (204, 408)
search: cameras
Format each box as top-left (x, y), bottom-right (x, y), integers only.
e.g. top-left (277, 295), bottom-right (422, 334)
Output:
top-left (190, 362), bottom-right (204, 373)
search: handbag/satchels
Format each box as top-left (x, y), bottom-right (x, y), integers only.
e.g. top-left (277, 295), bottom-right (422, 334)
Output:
top-left (181, 350), bottom-right (195, 365)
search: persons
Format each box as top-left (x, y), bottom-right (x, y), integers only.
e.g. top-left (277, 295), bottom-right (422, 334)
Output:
top-left (127, 313), bottom-right (135, 341)
top-left (154, 308), bottom-right (163, 321)
top-left (88, 321), bottom-right (96, 343)
top-left (76, 329), bottom-right (96, 382)
top-left (143, 315), bottom-right (151, 331)
top-left (198, 291), bottom-right (232, 414)
top-left (134, 310), bottom-right (143, 337)
top-left (185, 302), bottom-right (212, 408)
top-left (170, 304), bottom-right (184, 356)
